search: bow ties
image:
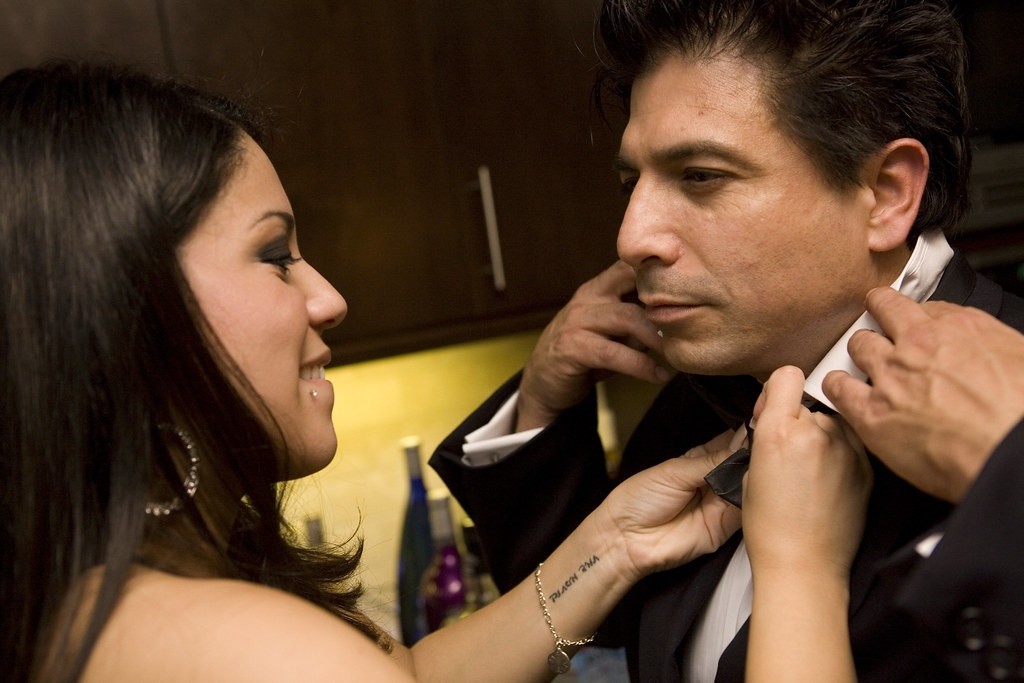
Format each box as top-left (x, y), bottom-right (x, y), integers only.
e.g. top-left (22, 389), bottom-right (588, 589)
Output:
top-left (703, 247), bottom-right (977, 510)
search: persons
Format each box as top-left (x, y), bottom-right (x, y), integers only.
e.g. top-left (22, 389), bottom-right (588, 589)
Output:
top-left (0, 63), bottom-right (868, 683)
top-left (422, 1), bottom-right (1024, 682)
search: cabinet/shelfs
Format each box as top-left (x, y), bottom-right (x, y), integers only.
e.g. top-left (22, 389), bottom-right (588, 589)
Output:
top-left (0, 0), bottom-right (629, 366)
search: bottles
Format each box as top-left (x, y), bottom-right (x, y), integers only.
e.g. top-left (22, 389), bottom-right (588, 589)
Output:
top-left (306, 513), bottom-right (325, 548)
top-left (396, 437), bottom-right (497, 648)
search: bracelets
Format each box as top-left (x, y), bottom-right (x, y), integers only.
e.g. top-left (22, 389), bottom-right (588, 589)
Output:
top-left (534, 562), bottom-right (598, 674)
